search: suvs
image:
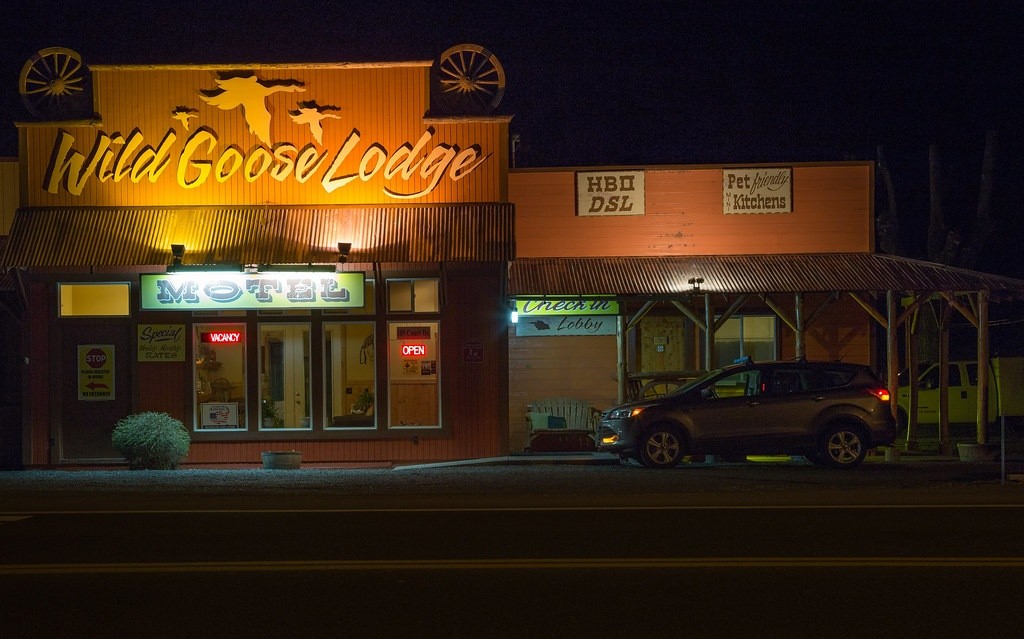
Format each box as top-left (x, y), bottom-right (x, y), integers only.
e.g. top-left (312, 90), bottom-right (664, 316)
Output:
top-left (596, 360), bottom-right (901, 469)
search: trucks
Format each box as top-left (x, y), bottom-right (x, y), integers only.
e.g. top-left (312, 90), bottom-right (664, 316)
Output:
top-left (883, 352), bottom-right (1024, 436)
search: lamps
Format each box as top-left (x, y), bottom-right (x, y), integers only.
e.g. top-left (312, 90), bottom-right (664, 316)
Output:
top-left (688, 278), bottom-right (704, 291)
top-left (170, 244), bottom-right (185, 265)
top-left (337, 242), bottom-right (351, 262)
